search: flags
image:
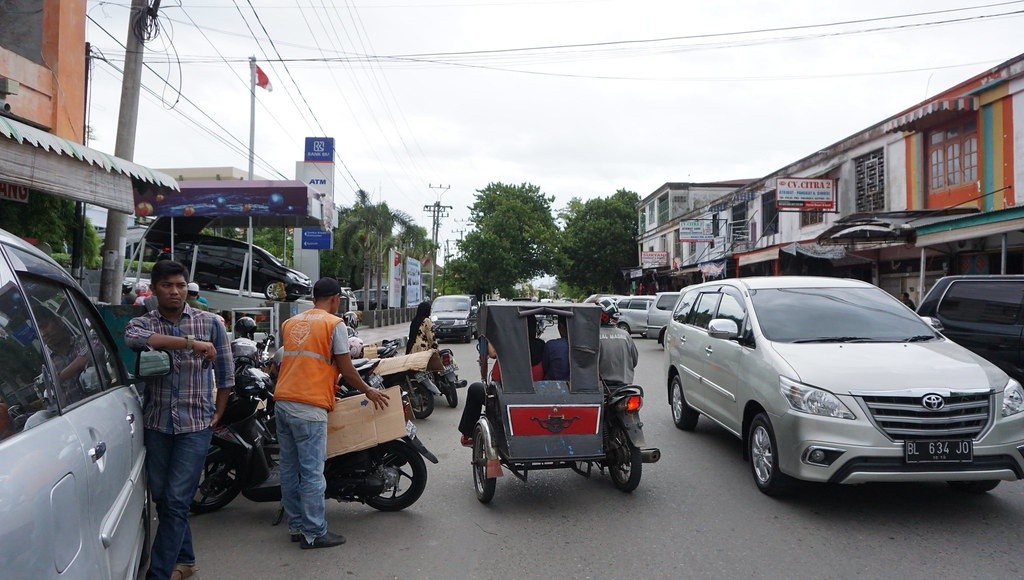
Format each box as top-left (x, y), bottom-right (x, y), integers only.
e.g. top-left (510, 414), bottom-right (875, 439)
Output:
top-left (256, 65), bottom-right (273, 91)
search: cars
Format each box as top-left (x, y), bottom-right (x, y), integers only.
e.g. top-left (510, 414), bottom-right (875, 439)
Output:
top-left (583, 290), bottom-right (678, 348)
top-left (663, 274), bottom-right (1024, 498)
top-left (0, 226), bottom-right (153, 580)
top-left (913, 274), bottom-right (1024, 390)
top-left (511, 297), bottom-right (574, 339)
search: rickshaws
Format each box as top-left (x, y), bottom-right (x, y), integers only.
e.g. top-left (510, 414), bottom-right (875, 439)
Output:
top-left (469, 300), bottom-right (662, 504)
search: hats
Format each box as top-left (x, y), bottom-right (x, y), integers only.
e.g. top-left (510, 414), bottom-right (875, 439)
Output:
top-left (314, 278), bottom-right (346, 297)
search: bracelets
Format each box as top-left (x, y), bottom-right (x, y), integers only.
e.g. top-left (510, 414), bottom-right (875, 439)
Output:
top-left (184, 335), bottom-right (194, 349)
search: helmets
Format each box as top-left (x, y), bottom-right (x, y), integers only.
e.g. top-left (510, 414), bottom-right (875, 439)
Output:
top-left (348, 337), bottom-right (364, 358)
top-left (235, 317), bottom-right (257, 336)
top-left (187, 283), bottom-right (199, 293)
top-left (231, 338), bottom-right (259, 368)
top-left (122, 281), bottom-right (133, 294)
top-left (596, 297), bottom-right (619, 320)
top-left (344, 312), bottom-right (358, 328)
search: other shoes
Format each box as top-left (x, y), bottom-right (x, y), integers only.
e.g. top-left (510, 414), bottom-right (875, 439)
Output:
top-left (461, 434), bottom-right (474, 448)
top-left (170, 563), bottom-right (197, 580)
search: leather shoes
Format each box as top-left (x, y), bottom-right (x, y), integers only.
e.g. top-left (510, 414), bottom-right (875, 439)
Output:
top-left (301, 529), bottom-right (345, 549)
top-left (291, 533), bottom-right (305, 542)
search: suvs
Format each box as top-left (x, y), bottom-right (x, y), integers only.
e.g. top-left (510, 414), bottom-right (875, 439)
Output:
top-left (429, 295), bottom-right (480, 344)
top-left (353, 289), bottom-right (388, 320)
top-left (156, 231), bottom-right (313, 302)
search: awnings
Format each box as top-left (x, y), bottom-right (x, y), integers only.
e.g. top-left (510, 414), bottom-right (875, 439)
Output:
top-left (0, 116), bottom-right (181, 192)
top-left (883, 95), bottom-right (980, 133)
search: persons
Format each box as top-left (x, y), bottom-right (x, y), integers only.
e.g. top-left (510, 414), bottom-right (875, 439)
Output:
top-left (134, 282), bottom-right (151, 305)
top-left (406, 302), bottom-right (439, 355)
top-left (458, 315), bottom-right (545, 447)
top-left (273, 277), bottom-right (389, 549)
top-left (188, 283), bottom-right (207, 305)
top-left (545, 316), bottom-right (569, 380)
top-left (900, 293), bottom-right (915, 311)
top-left (122, 281), bottom-right (135, 304)
top-left (124, 260), bottom-right (236, 580)
top-left (33, 307), bottom-right (105, 404)
top-left (597, 298), bottom-right (639, 386)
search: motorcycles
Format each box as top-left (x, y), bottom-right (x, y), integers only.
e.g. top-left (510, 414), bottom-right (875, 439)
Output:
top-left (189, 325), bottom-right (468, 527)
